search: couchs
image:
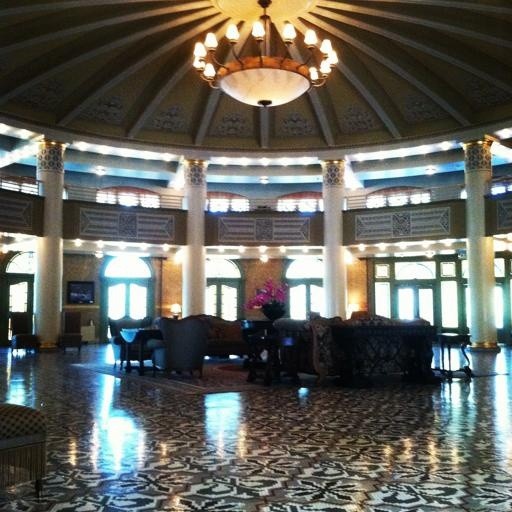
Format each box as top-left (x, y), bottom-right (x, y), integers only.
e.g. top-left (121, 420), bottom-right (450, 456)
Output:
top-left (107, 312), bottom-right (438, 383)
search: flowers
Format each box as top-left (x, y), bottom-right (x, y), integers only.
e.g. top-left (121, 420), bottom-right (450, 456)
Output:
top-left (239, 280), bottom-right (290, 310)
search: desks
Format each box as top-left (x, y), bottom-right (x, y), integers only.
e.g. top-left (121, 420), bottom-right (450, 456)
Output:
top-left (438, 332), bottom-right (472, 383)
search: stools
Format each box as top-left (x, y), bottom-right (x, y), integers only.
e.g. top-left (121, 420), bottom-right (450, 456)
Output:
top-left (0, 399), bottom-right (49, 504)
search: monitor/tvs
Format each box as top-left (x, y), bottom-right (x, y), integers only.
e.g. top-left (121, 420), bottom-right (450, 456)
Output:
top-left (67, 281), bottom-right (95, 304)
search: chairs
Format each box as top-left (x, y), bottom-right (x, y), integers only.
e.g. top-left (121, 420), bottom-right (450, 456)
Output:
top-left (62, 312), bottom-right (83, 356)
top-left (10, 313), bottom-right (40, 361)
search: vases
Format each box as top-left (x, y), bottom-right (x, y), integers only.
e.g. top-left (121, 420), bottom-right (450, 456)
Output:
top-left (259, 302), bottom-right (287, 323)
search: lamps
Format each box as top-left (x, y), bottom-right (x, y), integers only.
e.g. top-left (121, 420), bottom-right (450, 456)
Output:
top-left (190, 1), bottom-right (341, 110)
